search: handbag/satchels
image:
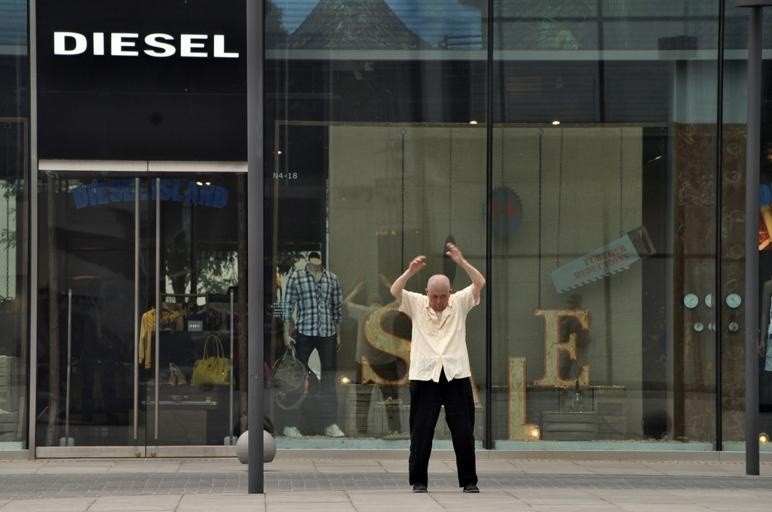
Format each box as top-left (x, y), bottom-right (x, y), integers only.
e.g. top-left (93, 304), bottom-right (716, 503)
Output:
top-left (273, 353), bottom-right (307, 394)
top-left (168, 363), bottom-right (188, 386)
top-left (192, 357), bottom-right (236, 386)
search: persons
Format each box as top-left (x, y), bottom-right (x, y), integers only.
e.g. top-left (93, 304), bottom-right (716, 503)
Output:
top-left (343, 274), bottom-right (402, 436)
top-left (282, 258), bottom-right (345, 437)
top-left (558, 293), bottom-right (588, 377)
top-left (389, 241), bottom-right (488, 492)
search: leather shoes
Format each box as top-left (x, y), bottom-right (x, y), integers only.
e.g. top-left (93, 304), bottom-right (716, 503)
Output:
top-left (377, 396), bottom-right (402, 405)
top-left (382, 430), bottom-right (410, 440)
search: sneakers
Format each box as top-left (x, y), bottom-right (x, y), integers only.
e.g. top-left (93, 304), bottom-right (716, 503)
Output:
top-left (413, 482), bottom-right (427, 493)
top-left (463, 484), bottom-right (479, 492)
top-left (282, 426), bottom-right (302, 439)
top-left (324, 424), bottom-right (345, 437)
